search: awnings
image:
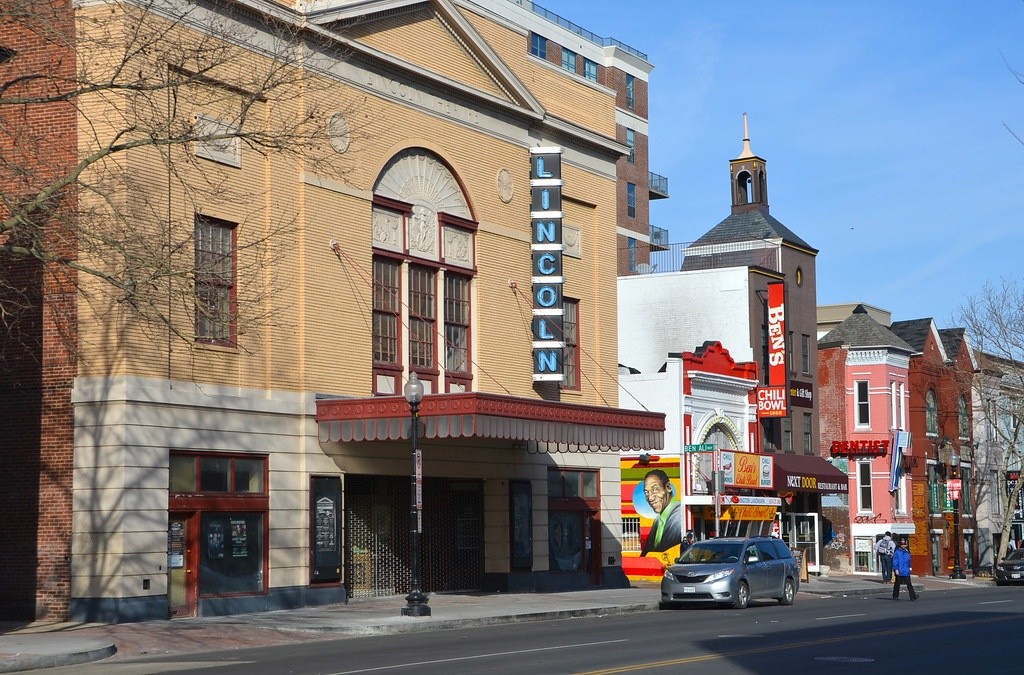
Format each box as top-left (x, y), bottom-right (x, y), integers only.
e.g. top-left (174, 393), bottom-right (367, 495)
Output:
top-left (766, 452), bottom-right (849, 494)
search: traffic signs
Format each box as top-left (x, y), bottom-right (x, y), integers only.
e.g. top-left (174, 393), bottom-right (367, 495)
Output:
top-left (684, 443), bottom-right (716, 452)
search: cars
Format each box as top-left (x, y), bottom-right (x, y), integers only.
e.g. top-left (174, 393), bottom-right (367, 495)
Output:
top-left (994, 547), bottom-right (1024, 586)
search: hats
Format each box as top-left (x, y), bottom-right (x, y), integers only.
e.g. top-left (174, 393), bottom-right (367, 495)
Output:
top-left (900, 537), bottom-right (906, 541)
top-left (687, 532), bottom-right (693, 538)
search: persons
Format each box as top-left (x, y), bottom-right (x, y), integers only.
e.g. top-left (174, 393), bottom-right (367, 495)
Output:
top-left (1008, 536), bottom-right (1017, 551)
top-left (892, 538), bottom-right (919, 601)
top-left (680, 533), bottom-right (693, 557)
top-left (874, 531), bottom-right (896, 585)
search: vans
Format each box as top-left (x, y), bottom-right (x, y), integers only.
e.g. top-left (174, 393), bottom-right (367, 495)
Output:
top-left (660, 536), bottom-right (800, 609)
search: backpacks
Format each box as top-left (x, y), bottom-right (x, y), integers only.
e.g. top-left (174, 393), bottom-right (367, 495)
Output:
top-left (877, 539), bottom-right (893, 555)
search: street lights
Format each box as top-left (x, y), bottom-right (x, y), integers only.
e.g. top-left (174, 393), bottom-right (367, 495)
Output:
top-left (401, 371), bottom-right (431, 616)
top-left (949, 449), bottom-right (967, 579)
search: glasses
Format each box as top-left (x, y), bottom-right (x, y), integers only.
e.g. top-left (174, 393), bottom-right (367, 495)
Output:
top-left (900, 542), bottom-right (906, 545)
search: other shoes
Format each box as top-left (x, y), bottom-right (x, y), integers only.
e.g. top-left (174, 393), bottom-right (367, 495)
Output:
top-left (893, 595), bottom-right (899, 601)
top-left (883, 579), bottom-right (886, 584)
top-left (887, 579), bottom-right (893, 584)
top-left (910, 594), bottom-right (919, 601)
